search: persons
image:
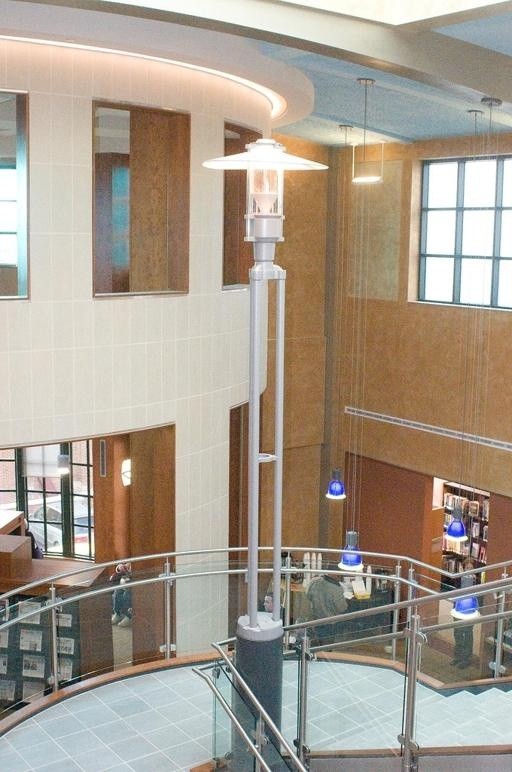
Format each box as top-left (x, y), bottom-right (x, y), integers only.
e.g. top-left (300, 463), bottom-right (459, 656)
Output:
top-left (294, 617), bottom-right (313, 639)
top-left (261, 591), bottom-right (284, 622)
top-left (24, 519), bottom-right (43, 560)
top-left (305, 563), bottom-right (349, 653)
top-left (448, 556), bottom-right (480, 669)
top-left (109, 562), bottom-right (133, 626)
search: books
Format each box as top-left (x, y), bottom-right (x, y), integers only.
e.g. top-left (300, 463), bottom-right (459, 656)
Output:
top-left (1, 598), bottom-right (77, 705)
top-left (441, 492), bottom-right (490, 584)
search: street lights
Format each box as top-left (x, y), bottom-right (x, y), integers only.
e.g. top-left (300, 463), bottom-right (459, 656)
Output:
top-left (197, 131), bottom-right (331, 772)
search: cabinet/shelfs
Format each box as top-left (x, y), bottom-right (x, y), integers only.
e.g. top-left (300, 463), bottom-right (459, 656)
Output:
top-left (1, 567), bottom-right (115, 707)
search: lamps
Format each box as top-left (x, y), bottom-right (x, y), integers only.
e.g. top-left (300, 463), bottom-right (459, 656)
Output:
top-left (202, 136), bottom-right (332, 262)
top-left (324, 77), bottom-right (366, 572)
top-left (443, 96), bottom-right (506, 618)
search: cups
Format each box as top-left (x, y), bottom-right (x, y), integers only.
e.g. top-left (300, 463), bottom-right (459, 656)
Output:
top-left (300, 551), bottom-right (323, 587)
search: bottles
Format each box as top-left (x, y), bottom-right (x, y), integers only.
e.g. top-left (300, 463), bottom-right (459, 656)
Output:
top-left (375, 568), bottom-right (387, 591)
top-left (281, 551), bottom-right (293, 580)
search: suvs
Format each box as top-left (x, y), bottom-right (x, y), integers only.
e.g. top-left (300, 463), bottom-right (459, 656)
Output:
top-left (22, 500), bottom-right (93, 555)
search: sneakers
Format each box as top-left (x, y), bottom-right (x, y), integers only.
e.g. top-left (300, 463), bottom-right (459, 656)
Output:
top-left (118, 615), bottom-right (132, 628)
top-left (111, 612), bottom-right (124, 625)
top-left (450, 657), bottom-right (472, 669)
